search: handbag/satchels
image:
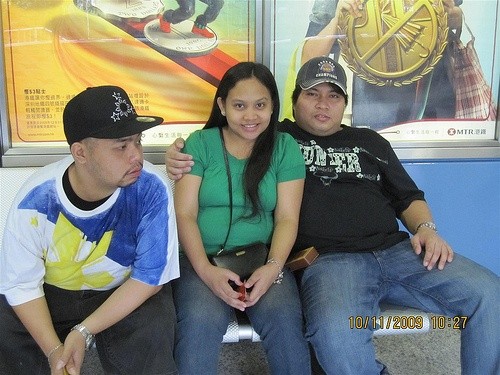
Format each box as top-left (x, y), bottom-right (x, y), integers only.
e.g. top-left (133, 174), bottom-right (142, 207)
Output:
top-left (211, 243), bottom-right (269, 289)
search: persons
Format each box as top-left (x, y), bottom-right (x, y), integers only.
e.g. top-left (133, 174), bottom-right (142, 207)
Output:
top-left (0, 87), bottom-right (182, 375)
top-left (165, 56), bottom-right (500, 375)
top-left (172, 60), bottom-right (312, 375)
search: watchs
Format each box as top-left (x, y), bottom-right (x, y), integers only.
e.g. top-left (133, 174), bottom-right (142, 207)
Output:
top-left (415, 222), bottom-right (438, 230)
top-left (70, 324), bottom-right (95, 352)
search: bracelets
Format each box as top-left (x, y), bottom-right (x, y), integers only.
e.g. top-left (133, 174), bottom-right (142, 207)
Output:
top-left (39, 341), bottom-right (64, 357)
top-left (268, 258), bottom-right (284, 284)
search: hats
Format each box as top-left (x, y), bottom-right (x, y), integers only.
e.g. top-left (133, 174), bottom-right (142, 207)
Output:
top-left (63, 85), bottom-right (164, 146)
top-left (296, 56), bottom-right (349, 96)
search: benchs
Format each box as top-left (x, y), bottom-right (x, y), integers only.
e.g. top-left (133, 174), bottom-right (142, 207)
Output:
top-left (80, 304), bottom-right (430, 375)
